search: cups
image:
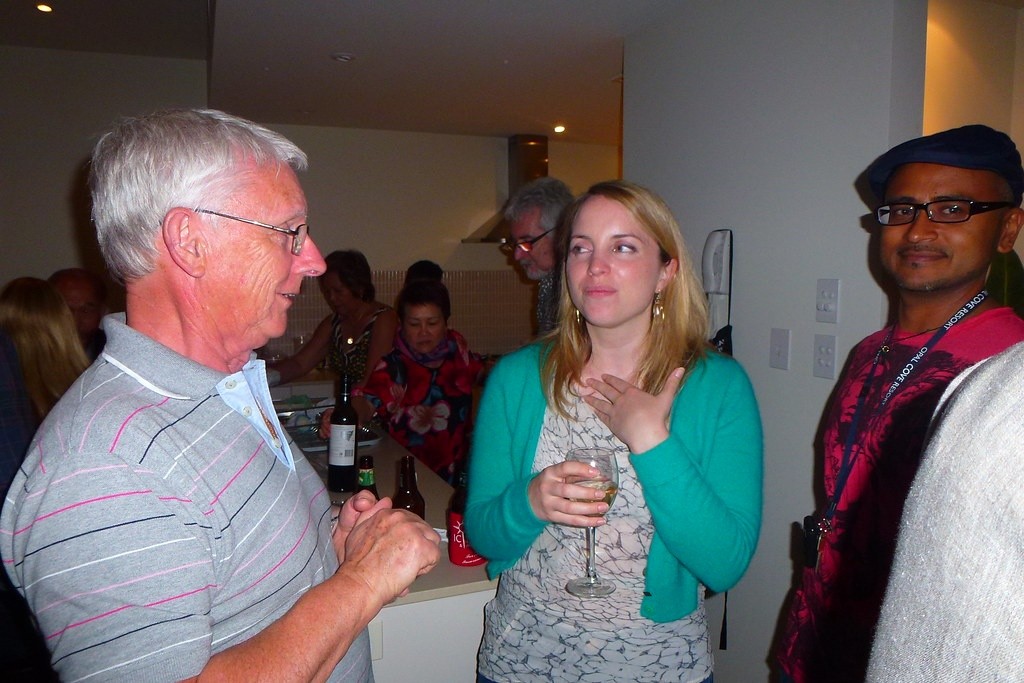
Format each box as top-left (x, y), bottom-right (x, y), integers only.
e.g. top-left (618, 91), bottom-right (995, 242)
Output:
top-left (294, 335), bottom-right (312, 354)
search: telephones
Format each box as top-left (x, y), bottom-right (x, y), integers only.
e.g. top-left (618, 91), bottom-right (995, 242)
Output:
top-left (701, 229), bottom-right (730, 295)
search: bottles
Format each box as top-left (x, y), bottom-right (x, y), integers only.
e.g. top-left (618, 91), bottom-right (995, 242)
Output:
top-left (391, 456), bottom-right (425, 522)
top-left (448, 461), bottom-right (489, 565)
top-left (353, 455), bottom-right (380, 502)
top-left (327, 371), bottom-right (358, 493)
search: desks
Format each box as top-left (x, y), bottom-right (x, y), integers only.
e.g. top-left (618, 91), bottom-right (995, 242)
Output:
top-left (282, 419), bottom-right (499, 683)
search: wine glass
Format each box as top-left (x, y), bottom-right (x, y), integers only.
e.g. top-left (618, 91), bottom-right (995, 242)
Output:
top-left (565, 447), bottom-right (619, 597)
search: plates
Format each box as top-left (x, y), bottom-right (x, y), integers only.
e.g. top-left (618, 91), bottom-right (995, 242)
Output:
top-left (292, 424), bottom-right (383, 452)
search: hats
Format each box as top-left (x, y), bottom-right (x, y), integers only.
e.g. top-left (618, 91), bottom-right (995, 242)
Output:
top-left (866, 124), bottom-right (1024, 207)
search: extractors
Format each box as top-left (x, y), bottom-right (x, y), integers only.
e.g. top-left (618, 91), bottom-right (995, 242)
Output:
top-left (461, 134), bottom-right (548, 244)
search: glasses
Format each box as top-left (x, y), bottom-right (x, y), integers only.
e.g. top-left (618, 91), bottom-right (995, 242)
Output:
top-left (873, 193), bottom-right (1018, 223)
top-left (497, 224), bottom-right (574, 254)
top-left (184, 207), bottom-right (310, 256)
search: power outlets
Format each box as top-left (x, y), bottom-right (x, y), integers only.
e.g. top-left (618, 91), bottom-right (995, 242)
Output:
top-left (768, 327), bottom-right (790, 371)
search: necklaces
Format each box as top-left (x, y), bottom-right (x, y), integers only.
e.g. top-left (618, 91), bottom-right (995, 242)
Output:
top-left (344, 336), bottom-right (354, 344)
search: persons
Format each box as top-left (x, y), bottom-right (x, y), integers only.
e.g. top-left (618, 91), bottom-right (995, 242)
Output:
top-left (319, 260), bottom-right (498, 482)
top-left (764, 123), bottom-right (1023, 683)
top-left (464, 180), bottom-right (763, 683)
top-left (0, 276), bottom-right (90, 507)
top-left (267, 250), bottom-right (398, 385)
top-left (865, 339), bottom-right (1024, 683)
top-left (47, 268), bottom-right (107, 363)
top-left (0, 109), bottom-right (441, 683)
top-left (502, 176), bottom-right (577, 330)
